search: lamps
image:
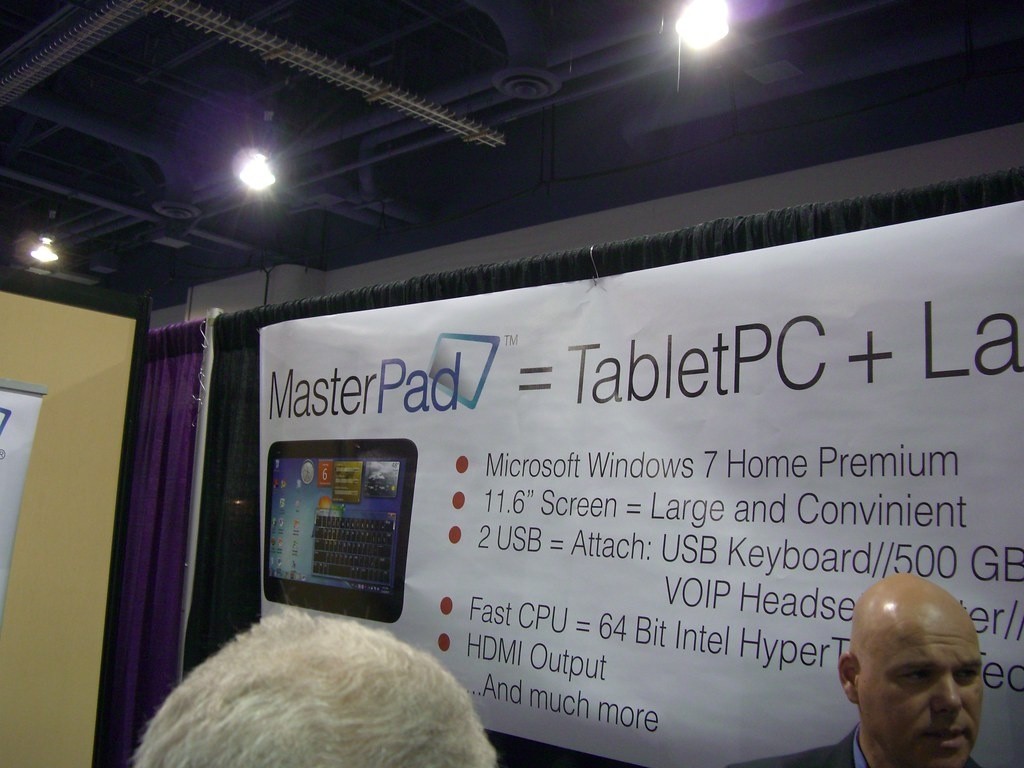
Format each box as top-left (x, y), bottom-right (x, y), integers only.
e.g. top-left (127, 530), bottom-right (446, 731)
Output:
top-left (32, 212), bottom-right (59, 266)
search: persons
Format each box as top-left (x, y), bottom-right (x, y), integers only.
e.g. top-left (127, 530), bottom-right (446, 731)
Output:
top-left (133, 608), bottom-right (498, 767)
top-left (728, 573), bottom-right (984, 768)
